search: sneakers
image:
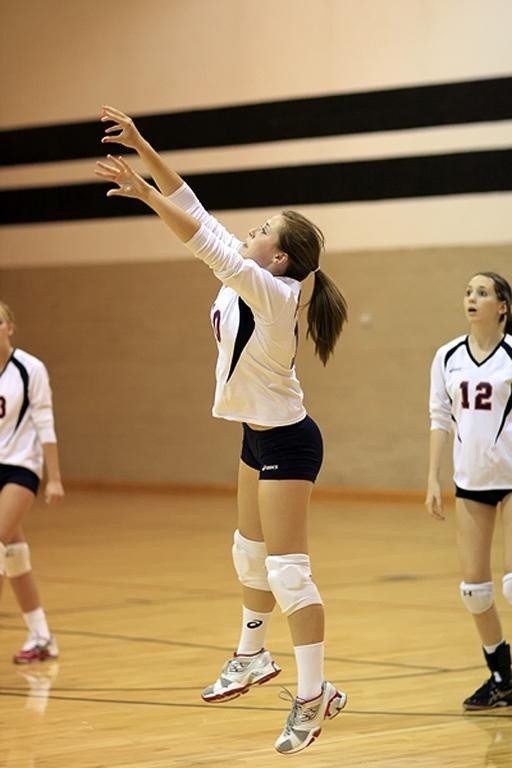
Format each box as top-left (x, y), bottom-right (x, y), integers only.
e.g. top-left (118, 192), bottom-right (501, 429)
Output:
top-left (13, 636), bottom-right (58, 663)
top-left (275, 684), bottom-right (347, 753)
top-left (201, 648), bottom-right (280, 704)
top-left (462, 673), bottom-right (512, 710)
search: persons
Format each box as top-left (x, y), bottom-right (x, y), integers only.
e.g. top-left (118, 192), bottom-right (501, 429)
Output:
top-left (90, 102), bottom-right (348, 755)
top-left (425, 270), bottom-right (511, 710)
top-left (1, 299), bottom-right (66, 664)
